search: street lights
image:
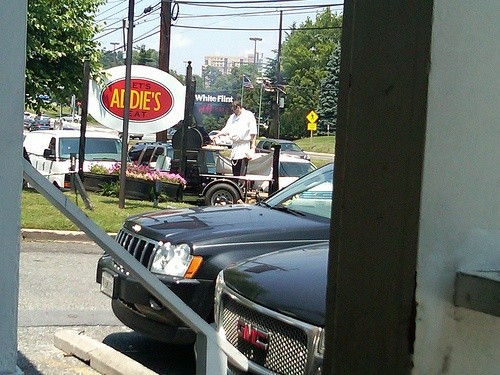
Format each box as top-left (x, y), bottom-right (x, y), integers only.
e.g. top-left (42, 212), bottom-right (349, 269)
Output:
top-left (111, 42), bottom-right (118, 54)
top-left (249, 37), bottom-right (262, 69)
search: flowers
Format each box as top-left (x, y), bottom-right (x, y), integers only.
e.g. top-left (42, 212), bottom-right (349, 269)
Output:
top-left (121, 160), bottom-right (187, 185)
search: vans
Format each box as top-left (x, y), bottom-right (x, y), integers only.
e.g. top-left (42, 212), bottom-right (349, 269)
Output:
top-left (23, 129), bottom-right (131, 191)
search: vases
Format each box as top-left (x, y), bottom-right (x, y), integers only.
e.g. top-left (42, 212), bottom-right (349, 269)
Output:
top-left (126, 176), bottom-right (184, 202)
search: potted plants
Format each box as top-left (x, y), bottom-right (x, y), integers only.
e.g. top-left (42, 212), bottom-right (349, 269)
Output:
top-left (83, 161), bottom-right (119, 193)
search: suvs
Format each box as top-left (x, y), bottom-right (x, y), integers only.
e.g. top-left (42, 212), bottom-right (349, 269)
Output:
top-left (132, 142), bottom-right (216, 176)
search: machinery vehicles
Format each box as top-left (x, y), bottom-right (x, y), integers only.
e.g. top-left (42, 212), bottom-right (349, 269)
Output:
top-left (156, 171), bottom-right (270, 207)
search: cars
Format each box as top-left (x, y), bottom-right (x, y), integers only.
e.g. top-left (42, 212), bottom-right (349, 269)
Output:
top-left (93, 161), bottom-right (339, 342)
top-left (270, 157), bottom-right (333, 201)
top-left (25, 91), bottom-right (84, 131)
top-left (257, 139), bottom-right (309, 163)
top-left (213, 241), bottom-right (330, 375)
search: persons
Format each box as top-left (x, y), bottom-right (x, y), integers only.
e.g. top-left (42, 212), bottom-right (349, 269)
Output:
top-left (212, 101), bottom-right (257, 177)
